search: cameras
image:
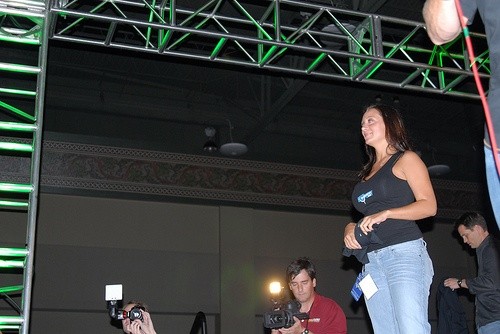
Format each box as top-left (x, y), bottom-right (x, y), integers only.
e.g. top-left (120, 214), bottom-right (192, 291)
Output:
top-left (104, 283), bottom-right (143, 323)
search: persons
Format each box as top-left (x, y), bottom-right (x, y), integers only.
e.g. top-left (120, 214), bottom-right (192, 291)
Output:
top-left (443, 211), bottom-right (500, 334)
top-left (343, 101), bottom-right (438, 334)
top-left (121, 301), bottom-right (158, 334)
top-left (271, 256), bottom-right (347, 334)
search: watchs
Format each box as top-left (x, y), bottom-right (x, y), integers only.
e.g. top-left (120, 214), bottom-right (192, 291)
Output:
top-left (458, 278), bottom-right (462, 289)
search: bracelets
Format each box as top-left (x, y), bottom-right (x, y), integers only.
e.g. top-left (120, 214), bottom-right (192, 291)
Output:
top-left (301, 328), bottom-right (309, 334)
